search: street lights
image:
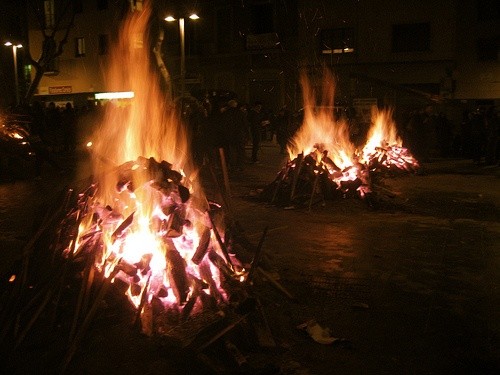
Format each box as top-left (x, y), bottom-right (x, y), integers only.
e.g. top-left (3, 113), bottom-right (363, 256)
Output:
top-left (164, 13), bottom-right (199, 90)
top-left (4, 41), bottom-right (24, 109)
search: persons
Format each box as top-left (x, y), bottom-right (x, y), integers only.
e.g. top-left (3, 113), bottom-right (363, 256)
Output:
top-left (164, 84), bottom-right (302, 170)
top-left (336, 93), bottom-right (499, 162)
top-left (25, 89), bottom-right (106, 168)
